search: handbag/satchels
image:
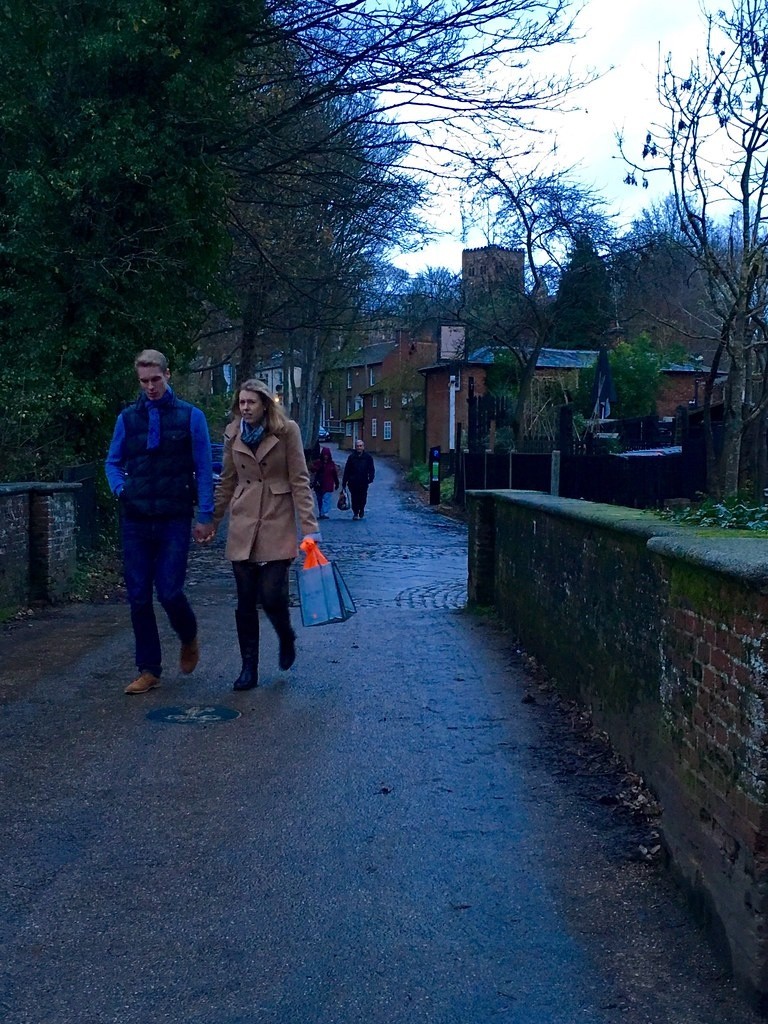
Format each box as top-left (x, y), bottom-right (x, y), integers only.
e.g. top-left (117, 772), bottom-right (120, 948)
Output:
top-left (295, 539), bottom-right (356, 626)
top-left (337, 489), bottom-right (350, 510)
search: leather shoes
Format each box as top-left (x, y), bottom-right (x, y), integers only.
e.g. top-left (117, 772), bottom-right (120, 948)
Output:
top-left (181, 635), bottom-right (199, 674)
top-left (124, 672), bottom-right (161, 693)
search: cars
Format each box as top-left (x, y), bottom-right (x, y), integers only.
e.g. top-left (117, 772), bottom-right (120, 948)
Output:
top-left (319, 424), bottom-right (330, 442)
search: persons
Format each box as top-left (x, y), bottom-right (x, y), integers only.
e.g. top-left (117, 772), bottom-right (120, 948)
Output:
top-left (106, 347), bottom-right (214, 695)
top-left (342, 440), bottom-right (375, 520)
top-left (195, 377), bottom-right (322, 691)
top-left (310, 446), bottom-right (340, 521)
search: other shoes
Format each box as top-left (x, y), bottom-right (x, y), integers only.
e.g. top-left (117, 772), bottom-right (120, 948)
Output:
top-left (321, 513), bottom-right (330, 518)
top-left (353, 515), bottom-right (358, 520)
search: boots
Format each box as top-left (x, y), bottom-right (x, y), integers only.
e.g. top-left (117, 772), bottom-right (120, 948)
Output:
top-left (234, 609), bottom-right (259, 691)
top-left (264, 601), bottom-right (296, 670)
